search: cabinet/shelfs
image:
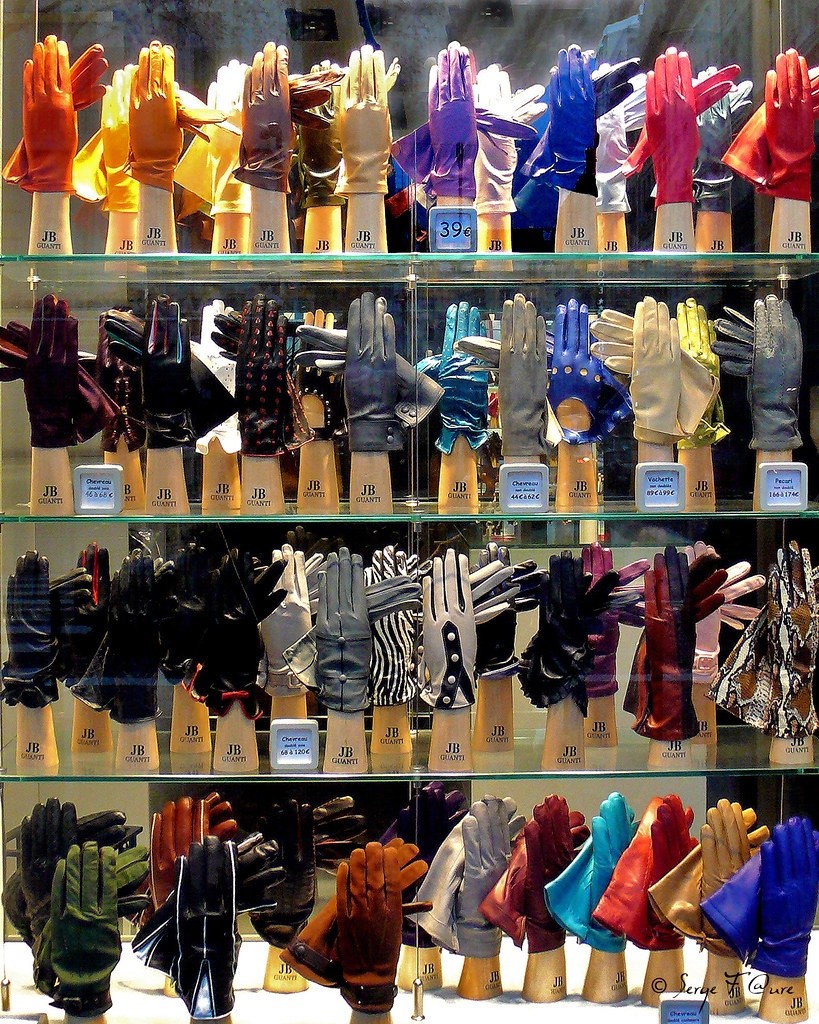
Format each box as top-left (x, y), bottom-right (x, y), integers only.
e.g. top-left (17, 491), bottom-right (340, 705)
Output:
top-left (0, 250), bottom-right (819, 1024)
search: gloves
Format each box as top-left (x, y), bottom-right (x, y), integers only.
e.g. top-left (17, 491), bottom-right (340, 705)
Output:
top-left (2, 781), bottom-right (819, 1020)
top-left (2, 34), bottom-right (819, 214)
top-left (0, 526), bottom-right (819, 738)
top-left (0, 292), bottom-right (802, 457)
